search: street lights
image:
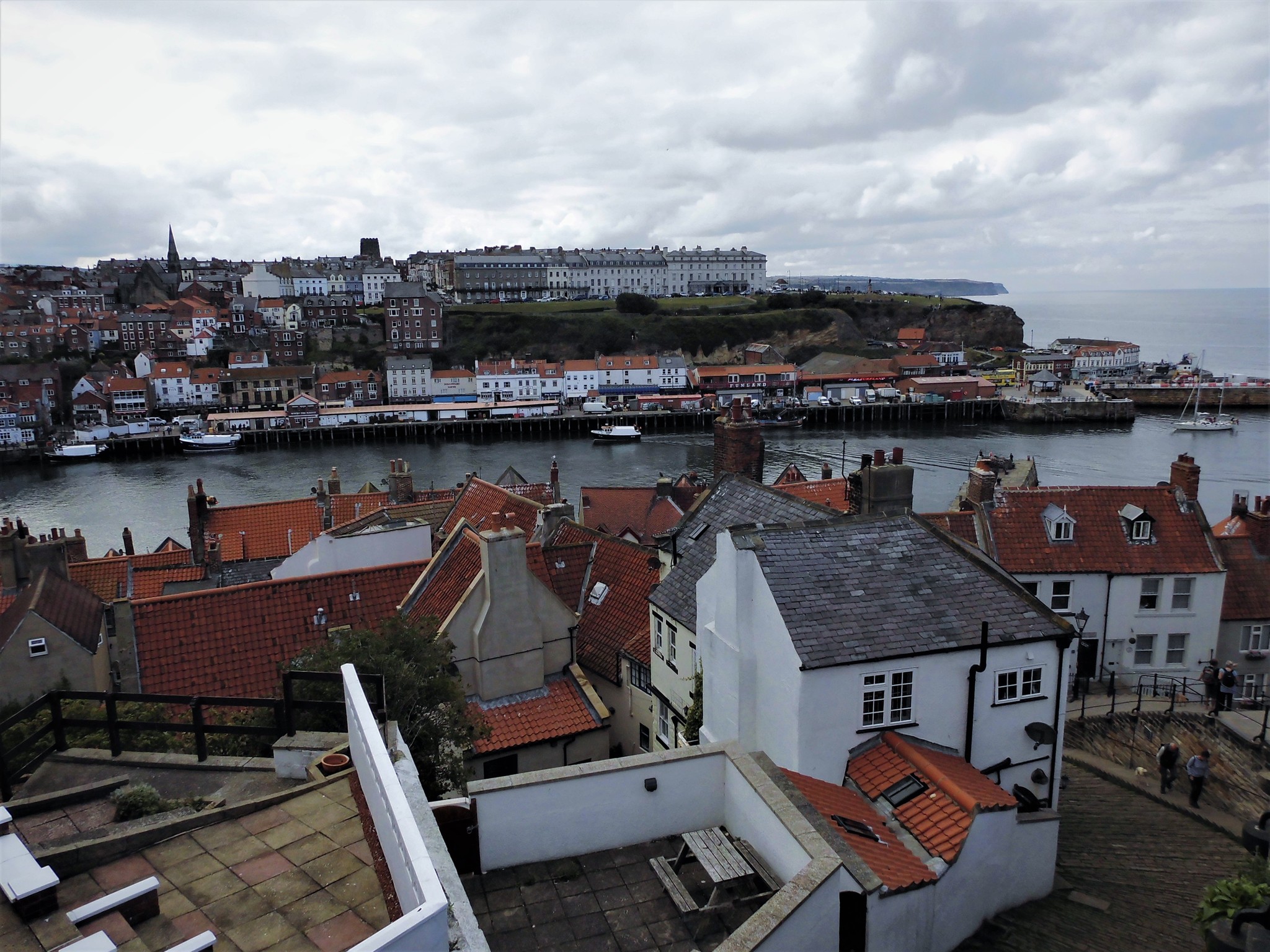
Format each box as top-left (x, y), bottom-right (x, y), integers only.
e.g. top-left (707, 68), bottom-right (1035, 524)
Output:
top-left (1074, 606), bottom-right (1089, 700)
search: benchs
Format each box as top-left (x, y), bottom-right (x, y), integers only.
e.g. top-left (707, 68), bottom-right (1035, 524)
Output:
top-left (0, 806), bottom-right (217, 952)
top-left (649, 856), bottom-right (699, 915)
top-left (733, 840), bottom-right (786, 892)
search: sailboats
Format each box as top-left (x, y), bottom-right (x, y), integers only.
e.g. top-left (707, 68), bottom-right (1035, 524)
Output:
top-left (1174, 349), bottom-right (1240, 431)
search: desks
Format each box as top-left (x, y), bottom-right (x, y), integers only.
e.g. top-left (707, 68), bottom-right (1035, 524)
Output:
top-left (662, 827), bottom-right (765, 941)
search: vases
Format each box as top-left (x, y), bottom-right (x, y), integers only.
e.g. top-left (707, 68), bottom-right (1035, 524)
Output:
top-left (321, 753), bottom-right (349, 774)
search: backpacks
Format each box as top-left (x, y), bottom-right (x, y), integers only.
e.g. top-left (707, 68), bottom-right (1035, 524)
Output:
top-left (1202, 665), bottom-right (1218, 685)
top-left (1221, 668), bottom-right (1236, 688)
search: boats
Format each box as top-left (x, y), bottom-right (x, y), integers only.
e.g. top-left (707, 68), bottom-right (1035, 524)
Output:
top-left (179, 430), bottom-right (242, 452)
top-left (43, 436), bottom-right (110, 463)
top-left (590, 423), bottom-right (642, 441)
top-left (756, 414), bottom-right (807, 429)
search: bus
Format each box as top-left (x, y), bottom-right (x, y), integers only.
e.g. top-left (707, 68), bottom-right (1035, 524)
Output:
top-left (982, 370), bottom-right (1017, 387)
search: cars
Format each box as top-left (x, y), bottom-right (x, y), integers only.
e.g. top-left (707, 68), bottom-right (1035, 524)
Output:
top-left (828, 397), bottom-right (841, 406)
top-left (849, 396), bottom-right (862, 405)
top-left (426, 280), bottom-right (909, 304)
top-left (1005, 348), bottom-right (1019, 353)
top-left (867, 338), bottom-right (910, 349)
top-left (989, 347), bottom-right (1003, 352)
top-left (800, 399), bottom-right (809, 408)
top-left (972, 346), bottom-right (989, 352)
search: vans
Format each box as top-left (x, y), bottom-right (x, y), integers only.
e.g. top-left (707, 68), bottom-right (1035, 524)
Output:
top-left (144, 417), bottom-right (167, 426)
top-left (818, 396), bottom-right (829, 406)
top-left (791, 397), bottom-right (800, 407)
top-left (1173, 374), bottom-right (1193, 384)
top-left (583, 402), bottom-right (612, 414)
top-left (751, 399), bottom-right (761, 410)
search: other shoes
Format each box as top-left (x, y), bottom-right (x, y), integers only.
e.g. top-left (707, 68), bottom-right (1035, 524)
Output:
top-left (1188, 798), bottom-right (1200, 809)
top-left (1165, 778), bottom-right (1172, 789)
top-left (1160, 787), bottom-right (1166, 794)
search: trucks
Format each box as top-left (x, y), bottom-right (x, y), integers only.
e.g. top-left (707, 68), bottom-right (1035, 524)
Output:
top-left (881, 388), bottom-right (902, 397)
top-left (865, 389), bottom-right (876, 403)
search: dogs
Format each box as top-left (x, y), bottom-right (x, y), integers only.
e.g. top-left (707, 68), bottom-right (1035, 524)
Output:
top-left (1134, 767), bottom-right (1150, 787)
top-left (1168, 692), bottom-right (1189, 707)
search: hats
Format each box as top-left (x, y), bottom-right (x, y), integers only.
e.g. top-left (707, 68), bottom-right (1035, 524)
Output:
top-left (1225, 660), bottom-right (1233, 666)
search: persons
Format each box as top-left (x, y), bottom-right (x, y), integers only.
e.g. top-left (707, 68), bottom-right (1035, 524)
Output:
top-left (1198, 659), bottom-right (1238, 710)
top-left (1157, 743), bottom-right (1181, 794)
top-left (1187, 750), bottom-right (1210, 808)
top-left (1218, 661), bottom-right (1239, 711)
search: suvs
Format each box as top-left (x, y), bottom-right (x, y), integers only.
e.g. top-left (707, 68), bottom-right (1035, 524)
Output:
top-left (1021, 348), bottom-right (1035, 354)
top-left (172, 417), bottom-right (179, 425)
top-left (1038, 350), bottom-right (1051, 355)
